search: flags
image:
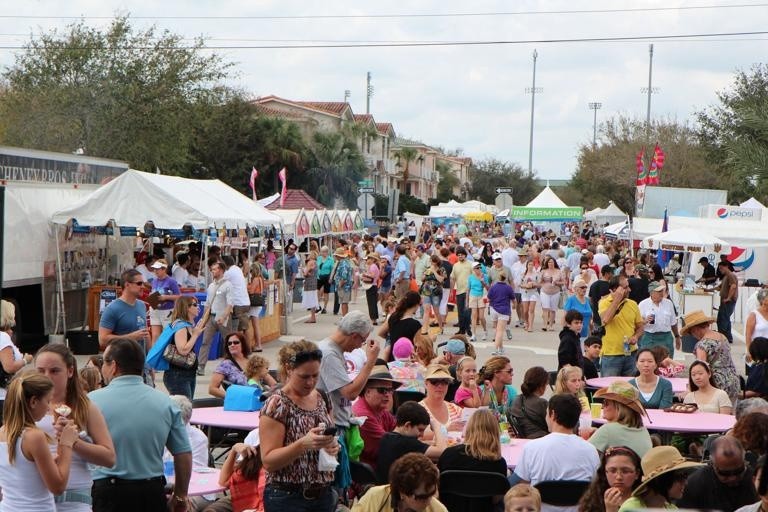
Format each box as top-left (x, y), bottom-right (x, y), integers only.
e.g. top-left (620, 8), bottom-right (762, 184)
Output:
top-left (249, 167), bottom-right (258, 202)
top-left (637, 145), bottom-right (646, 185)
top-left (657, 207), bottom-right (670, 271)
top-left (645, 143), bottom-right (664, 185)
top-left (279, 166), bottom-right (288, 207)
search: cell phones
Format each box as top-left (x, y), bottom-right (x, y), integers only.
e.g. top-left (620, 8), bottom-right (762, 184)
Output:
top-left (324, 428), bottom-right (337, 437)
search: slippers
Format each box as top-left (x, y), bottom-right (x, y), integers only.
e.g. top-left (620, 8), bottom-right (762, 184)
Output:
top-left (250, 344), bottom-right (263, 352)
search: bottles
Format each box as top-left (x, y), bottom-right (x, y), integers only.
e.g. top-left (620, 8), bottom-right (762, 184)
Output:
top-left (648, 308), bottom-right (656, 325)
top-left (624, 337), bottom-right (631, 356)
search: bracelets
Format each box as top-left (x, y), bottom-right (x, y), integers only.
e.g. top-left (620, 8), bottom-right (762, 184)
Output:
top-left (21, 358), bottom-right (29, 369)
top-left (170, 491), bottom-right (189, 505)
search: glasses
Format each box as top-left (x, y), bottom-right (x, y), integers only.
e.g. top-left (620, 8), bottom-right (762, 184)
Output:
top-left (99, 358), bottom-right (112, 367)
top-left (288, 246), bottom-right (296, 251)
top-left (493, 258), bottom-right (502, 261)
top-left (367, 385), bottom-right (396, 395)
top-left (415, 250), bottom-right (420, 253)
top-left (215, 253), bottom-right (221, 256)
top-left (427, 379), bottom-right (451, 385)
top-left (443, 350), bottom-right (447, 356)
top-left (582, 267), bottom-right (589, 270)
top-left (188, 303), bottom-right (197, 307)
top-left (605, 469), bottom-right (638, 477)
top-left (647, 270), bottom-right (655, 274)
top-left (486, 246), bottom-right (492, 248)
top-left (610, 271), bottom-right (614, 275)
top-left (667, 472), bottom-right (689, 484)
top-left (359, 335), bottom-right (367, 347)
top-left (408, 484), bottom-right (438, 501)
top-left (714, 463), bottom-right (746, 477)
top-left (602, 399), bottom-right (611, 407)
top-left (128, 280), bottom-right (145, 286)
top-left (287, 350), bottom-right (322, 364)
top-left (473, 266), bottom-right (482, 269)
top-left (499, 367), bottom-right (514, 374)
top-left (226, 341), bottom-right (241, 345)
top-left (435, 243), bottom-right (438, 246)
top-left (578, 285), bottom-right (588, 289)
top-left (260, 257), bottom-right (266, 260)
top-left (625, 262), bottom-right (633, 264)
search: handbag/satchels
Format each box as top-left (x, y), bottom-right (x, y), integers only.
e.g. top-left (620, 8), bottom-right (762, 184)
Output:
top-left (249, 293), bottom-right (266, 306)
top-left (145, 321), bottom-right (172, 372)
top-left (162, 344), bottom-right (197, 369)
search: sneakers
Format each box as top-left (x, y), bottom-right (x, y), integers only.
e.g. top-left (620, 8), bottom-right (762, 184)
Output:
top-left (470, 337), bottom-right (477, 341)
top-left (421, 332), bottom-right (429, 336)
top-left (542, 326), bottom-right (547, 331)
top-left (492, 351), bottom-right (498, 355)
top-left (515, 320), bottom-right (524, 329)
top-left (493, 337), bottom-right (496, 341)
top-left (529, 328), bottom-right (533, 332)
top-left (315, 306), bottom-right (321, 313)
top-left (382, 312), bottom-right (386, 317)
top-left (482, 334), bottom-right (487, 340)
top-left (524, 323), bottom-right (528, 330)
top-left (304, 319), bottom-right (316, 324)
top-left (321, 308), bottom-right (327, 314)
top-left (549, 326), bottom-right (554, 331)
top-left (455, 332), bottom-right (465, 335)
top-left (453, 323), bottom-right (460, 327)
top-left (195, 369), bottom-right (205, 376)
top-left (508, 334), bottom-right (513, 340)
top-left (499, 351), bottom-right (505, 355)
top-left (373, 322), bottom-right (378, 326)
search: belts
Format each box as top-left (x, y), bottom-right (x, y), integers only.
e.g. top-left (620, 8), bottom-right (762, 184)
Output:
top-left (265, 477), bottom-right (336, 502)
top-left (92, 474), bottom-right (166, 489)
top-left (48, 488), bottom-right (96, 505)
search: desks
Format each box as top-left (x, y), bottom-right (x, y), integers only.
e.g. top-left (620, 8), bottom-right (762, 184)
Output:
top-left (675, 283), bottom-right (713, 333)
top-left (190, 406), bottom-right (262, 456)
top-left (163, 461), bottom-right (231, 499)
top-left (588, 402), bottom-right (736, 437)
top-left (585, 376), bottom-right (691, 394)
top-left (88, 277), bottom-right (282, 363)
top-left (500, 435), bottom-right (533, 471)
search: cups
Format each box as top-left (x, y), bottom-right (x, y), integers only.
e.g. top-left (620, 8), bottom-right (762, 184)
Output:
top-left (578, 396), bottom-right (590, 413)
top-left (591, 403), bottom-right (602, 419)
top-left (162, 461), bottom-right (175, 476)
top-left (551, 281), bottom-right (556, 287)
top-left (528, 282), bottom-right (532, 288)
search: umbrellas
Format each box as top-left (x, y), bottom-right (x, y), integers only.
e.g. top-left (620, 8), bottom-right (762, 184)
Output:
top-left (637, 227), bottom-right (730, 252)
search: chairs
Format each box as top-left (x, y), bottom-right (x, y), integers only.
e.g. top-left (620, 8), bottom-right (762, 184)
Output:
top-left (389, 389), bottom-right (425, 414)
top-left (343, 455), bottom-right (376, 507)
top-left (549, 371), bottom-right (558, 392)
top-left (532, 479), bottom-right (592, 508)
top-left (261, 367), bottom-right (282, 400)
top-left (438, 468), bottom-right (511, 512)
top-left (194, 398), bottom-right (247, 462)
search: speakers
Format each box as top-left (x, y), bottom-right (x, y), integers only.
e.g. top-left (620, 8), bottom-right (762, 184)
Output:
top-left (64, 330), bottom-right (99, 355)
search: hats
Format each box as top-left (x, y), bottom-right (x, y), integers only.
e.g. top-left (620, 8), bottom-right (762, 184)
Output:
top-left (423, 364), bottom-right (454, 380)
top-left (518, 250), bottom-right (528, 256)
top-left (386, 237), bottom-right (396, 243)
top-left (698, 257), bottom-right (708, 263)
top-left (416, 244), bottom-right (425, 253)
top-left (647, 281), bottom-right (666, 293)
top-left (332, 248), bottom-right (349, 258)
top-left (592, 381), bottom-right (646, 417)
top-left (367, 252), bottom-right (381, 261)
top-left (380, 253), bottom-right (391, 263)
top-left (630, 445), bottom-right (708, 498)
top-left (446, 339), bottom-right (466, 355)
top-left (359, 364), bottom-right (403, 396)
top-left (491, 253), bottom-right (502, 260)
top-left (601, 264), bottom-right (616, 274)
top-left (679, 309), bottom-right (716, 336)
top-left (392, 337), bottom-right (414, 359)
top-left (471, 262), bottom-right (481, 269)
top-left (150, 261), bottom-right (168, 269)
top-left (235, 427), bottom-right (260, 462)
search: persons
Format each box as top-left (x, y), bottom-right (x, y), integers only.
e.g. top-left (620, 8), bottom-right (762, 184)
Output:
top-left (130, 236), bottom-right (270, 284)
top-left (79, 368), bottom-right (100, 393)
top-left (148, 260), bottom-right (182, 345)
top-left (391, 216), bottom-right (511, 330)
top-left (202, 429), bottom-right (266, 511)
top-left (99, 270), bottom-right (155, 385)
top-left (34, 343), bottom-right (116, 510)
top-left (162, 296), bottom-right (206, 403)
top-left (209, 334), bottom-right (276, 398)
top-left (315, 312), bottom-right (380, 494)
top-left (84, 356), bottom-right (106, 389)
top-left (628, 244), bottom-right (768, 512)
top-left (512, 218), bottom-right (628, 330)
top-left (194, 263), bottom-right (233, 375)
top-left (221, 256), bottom-right (252, 355)
top-left (247, 356), bottom-right (272, 399)
top-left (270, 234), bottom-right (391, 325)
top-left (351, 330), bottom-right (628, 512)
top-left (0, 376), bottom-right (79, 510)
top-left (87, 339), bottom-right (193, 510)
top-left (248, 261), bottom-right (263, 351)
top-left (0, 300), bottom-right (33, 424)
top-left (260, 340), bottom-right (342, 512)
